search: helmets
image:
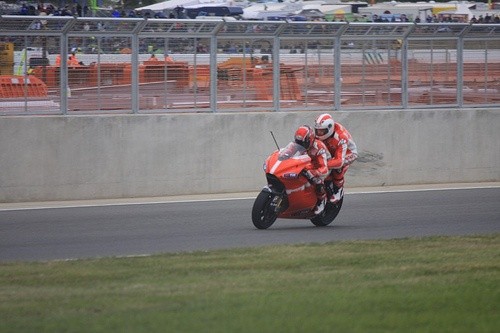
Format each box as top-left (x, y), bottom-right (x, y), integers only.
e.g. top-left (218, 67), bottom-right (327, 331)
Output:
top-left (314, 114), bottom-right (334, 140)
top-left (294, 127), bottom-right (313, 150)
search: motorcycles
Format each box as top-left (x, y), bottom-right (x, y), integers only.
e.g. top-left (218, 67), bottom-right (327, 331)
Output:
top-left (251, 148), bottom-right (344, 229)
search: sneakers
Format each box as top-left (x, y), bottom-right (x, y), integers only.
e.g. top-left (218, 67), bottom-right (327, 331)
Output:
top-left (313, 193), bottom-right (328, 215)
top-left (329, 187), bottom-right (344, 203)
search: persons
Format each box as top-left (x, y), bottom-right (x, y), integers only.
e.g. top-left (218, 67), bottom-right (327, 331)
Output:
top-left (314, 114), bottom-right (357, 203)
top-left (0, 0), bottom-right (500, 67)
top-left (280, 124), bottom-right (333, 216)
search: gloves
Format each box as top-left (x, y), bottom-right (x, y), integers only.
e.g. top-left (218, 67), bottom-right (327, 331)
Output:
top-left (305, 168), bottom-right (320, 183)
